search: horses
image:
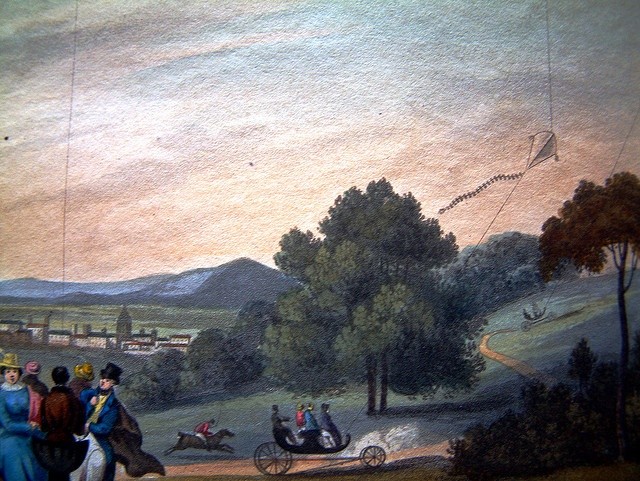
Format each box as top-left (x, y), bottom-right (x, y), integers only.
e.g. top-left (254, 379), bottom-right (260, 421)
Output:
top-left (162, 428), bottom-right (235, 457)
